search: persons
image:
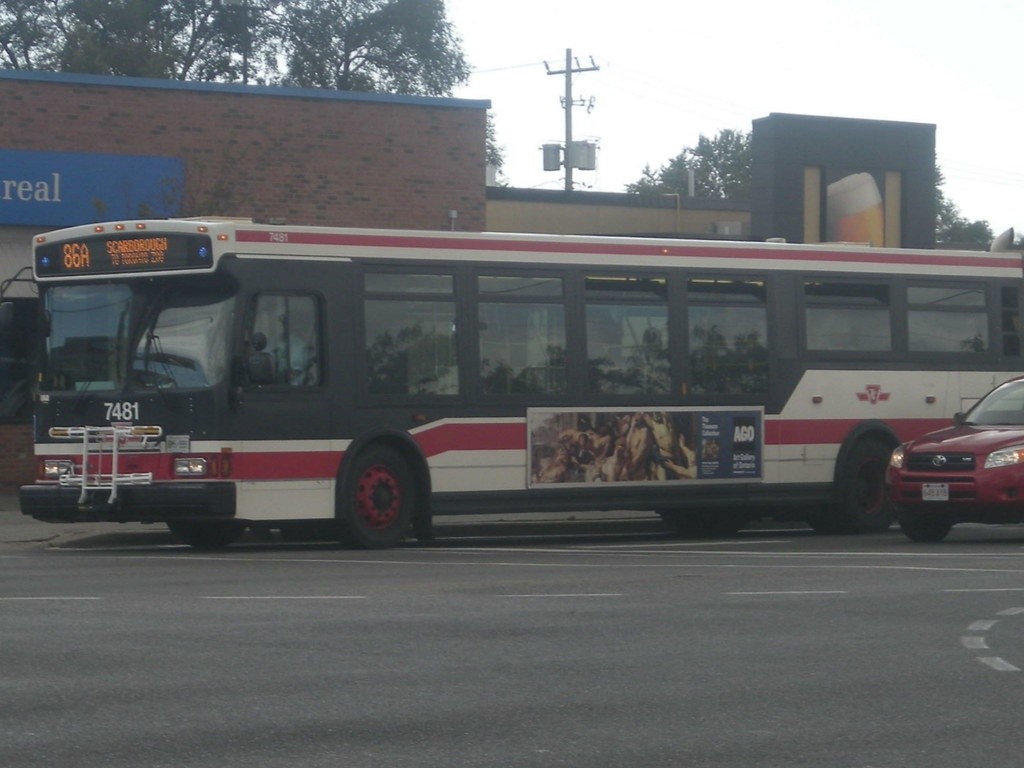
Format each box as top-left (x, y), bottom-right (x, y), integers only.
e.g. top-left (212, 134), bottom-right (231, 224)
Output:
top-left (532, 412), bottom-right (719, 483)
top-left (633, 326), bottom-right (672, 397)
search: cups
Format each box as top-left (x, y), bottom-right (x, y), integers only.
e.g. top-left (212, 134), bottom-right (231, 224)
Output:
top-left (827, 181), bottom-right (885, 247)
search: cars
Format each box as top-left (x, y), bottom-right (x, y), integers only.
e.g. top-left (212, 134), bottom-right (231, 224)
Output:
top-left (882, 376), bottom-right (1024, 541)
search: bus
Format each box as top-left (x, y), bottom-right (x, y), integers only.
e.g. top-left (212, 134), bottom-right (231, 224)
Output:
top-left (0, 213), bottom-right (1024, 546)
